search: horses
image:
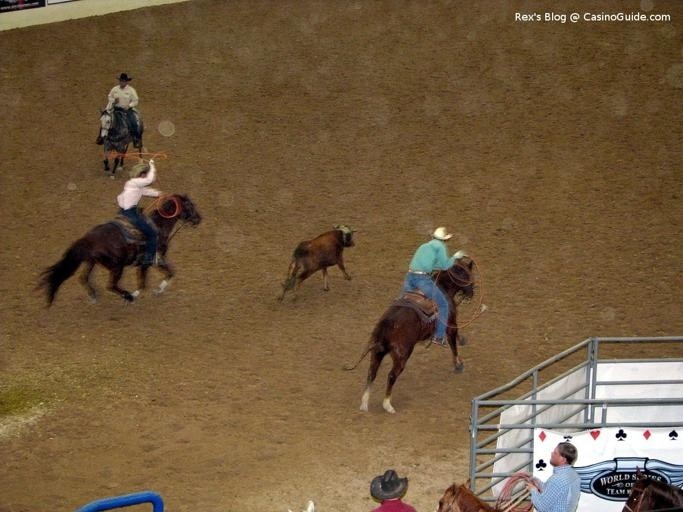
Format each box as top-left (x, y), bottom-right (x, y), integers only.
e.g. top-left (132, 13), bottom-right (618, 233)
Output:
top-left (434, 478), bottom-right (503, 512)
top-left (98, 106), bottom-right (144, 181)
top-left (342, 258), bottom-right (475, 415)
top-left (622, 466), bottom-right (683, 512)
top-left (33, 193), bottom-right (202, 306)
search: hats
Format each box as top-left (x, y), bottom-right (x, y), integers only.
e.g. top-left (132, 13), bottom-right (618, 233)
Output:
top-left (372, 470), bottom-right (408, 499)
top-left (128, 161), bottom-right (150, 180)
top-left (115, 73), bottom-right (132, 82)
top-left (430, 226), bottom-right (454, 241)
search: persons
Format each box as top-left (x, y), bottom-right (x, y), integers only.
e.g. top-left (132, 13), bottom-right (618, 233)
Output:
top-left (400, 227), bottom-right (466, 347)
top-left (370, 469), bottom-right (416, 511)
top-left (116, 160), bottom-right (163, 266)
top-left (95, 73), bottom-right (141, 148)
top-left (525, 442), bottom-right (581, 511)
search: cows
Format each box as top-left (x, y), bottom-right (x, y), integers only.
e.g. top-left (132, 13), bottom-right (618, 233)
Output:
top-left (277, 223), bottom-right (357, 303)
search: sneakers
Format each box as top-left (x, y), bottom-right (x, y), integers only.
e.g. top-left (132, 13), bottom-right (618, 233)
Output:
top-left (430, 334), bottom-right (450, 350)
